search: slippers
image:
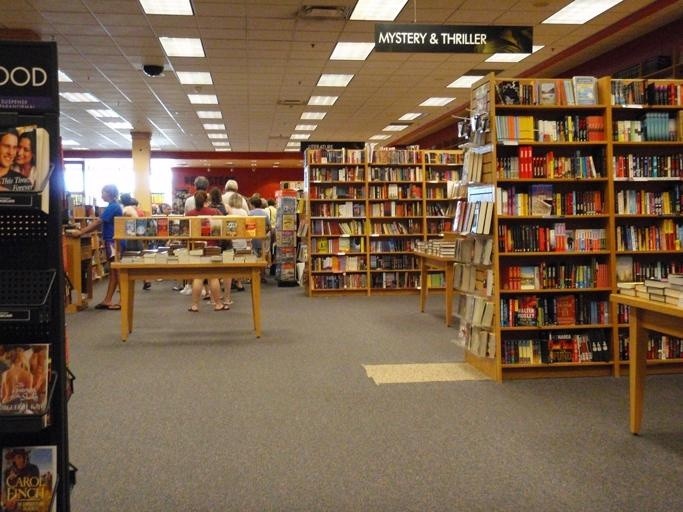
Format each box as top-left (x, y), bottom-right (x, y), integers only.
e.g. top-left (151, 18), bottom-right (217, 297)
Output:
top-left (188, 307), bottom-right (199, 312)
top-left (94, 303), bottom-right (112, 310)
top-left (214, 305), bottom-right (229, 311)
top-left (107, 304), bottom-right (121, 310)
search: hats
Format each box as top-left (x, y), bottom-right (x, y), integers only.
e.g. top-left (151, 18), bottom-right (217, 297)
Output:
top-left (5, 449), bottom-right (31, 460)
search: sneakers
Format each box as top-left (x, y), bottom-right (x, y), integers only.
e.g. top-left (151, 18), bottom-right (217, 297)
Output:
top-left (179, 285), bottom-right (191, 295)
top-left (142, 282), bottom-right (151, 289)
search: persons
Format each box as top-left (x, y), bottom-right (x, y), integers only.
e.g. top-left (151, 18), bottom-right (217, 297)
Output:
top-left (4, 448), bottom-right (41, 498)
top-left (1, 345), bottom-right (33, 407)
top-left (10, 127), bottom-right (42, 195)
top-left (28, 348), bottom-right (48, 410)
top-left (0, 126), bottom-right (32, 193)
top-left (1, 345), bottom-right (12, 375)
top-left (64, 172), bottom-right (279, 314)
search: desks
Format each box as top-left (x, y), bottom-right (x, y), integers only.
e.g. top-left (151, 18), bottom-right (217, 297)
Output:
top-left (609, 293), bottom-right (683, 435)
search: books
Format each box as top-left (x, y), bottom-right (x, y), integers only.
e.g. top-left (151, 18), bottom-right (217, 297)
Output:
top-left (453, 86), bottom-right (495, 359)
top-left (0, 342), bottom-right (54, 429)
top-left (276, 148), bottom-right (454, 292)
top-left (495, 77), bottom-right (612, 365)
top-left (0, 444), bottom-right (59, 511)
top-left (0, 123), bottom-right (52, 216)
top-left (612, 77), bottom-right (682, 359)
